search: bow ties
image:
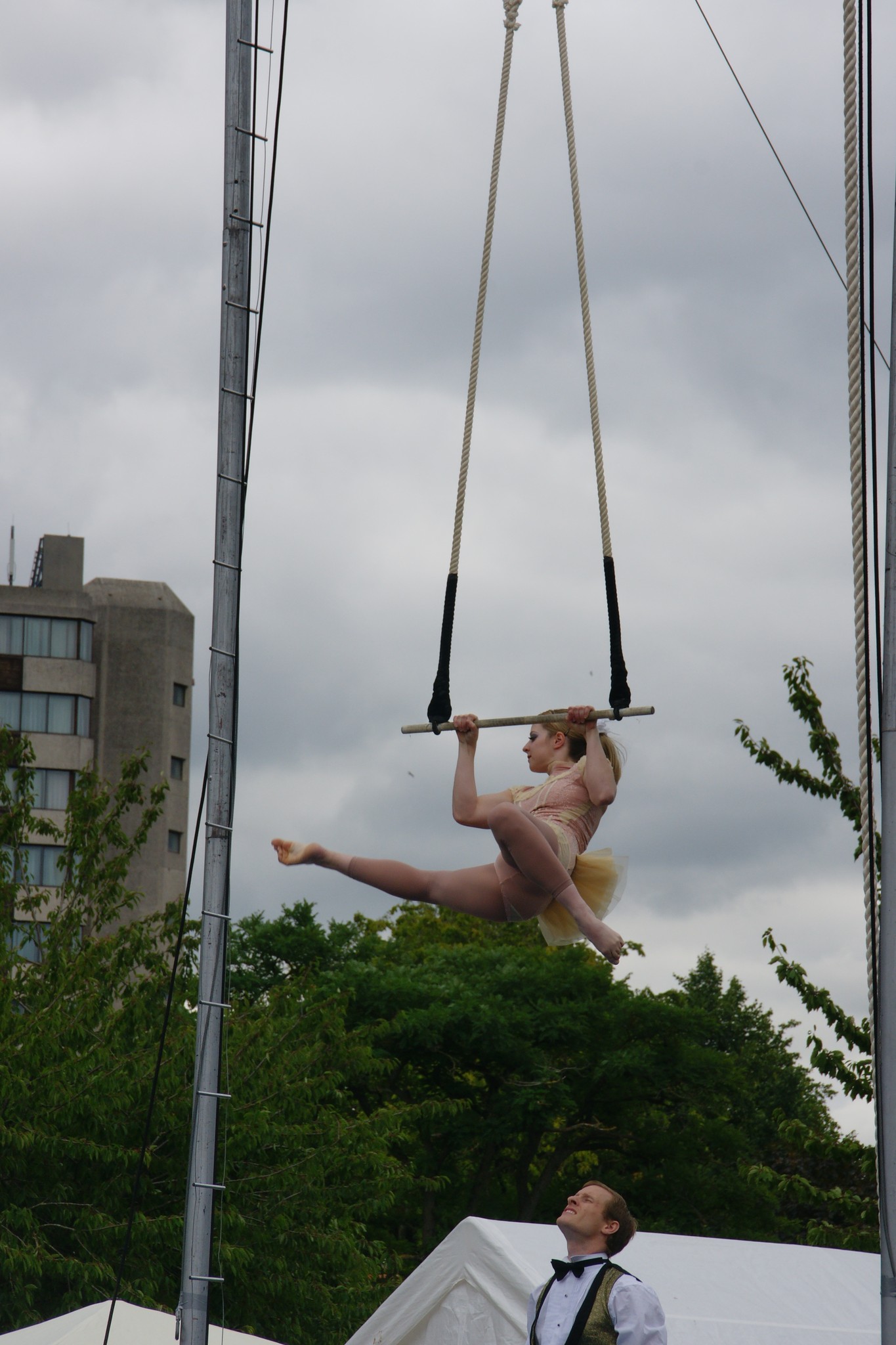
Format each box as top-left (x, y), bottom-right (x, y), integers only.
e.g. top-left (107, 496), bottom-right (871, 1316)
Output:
top-left (551, 1258), bottom-right (610, 1282)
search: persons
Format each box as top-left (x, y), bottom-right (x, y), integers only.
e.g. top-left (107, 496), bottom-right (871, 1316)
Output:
top-left (271, 706), bottom-right (626, 966)
top-left (526, 1179), bottom-right (667, 1345)
